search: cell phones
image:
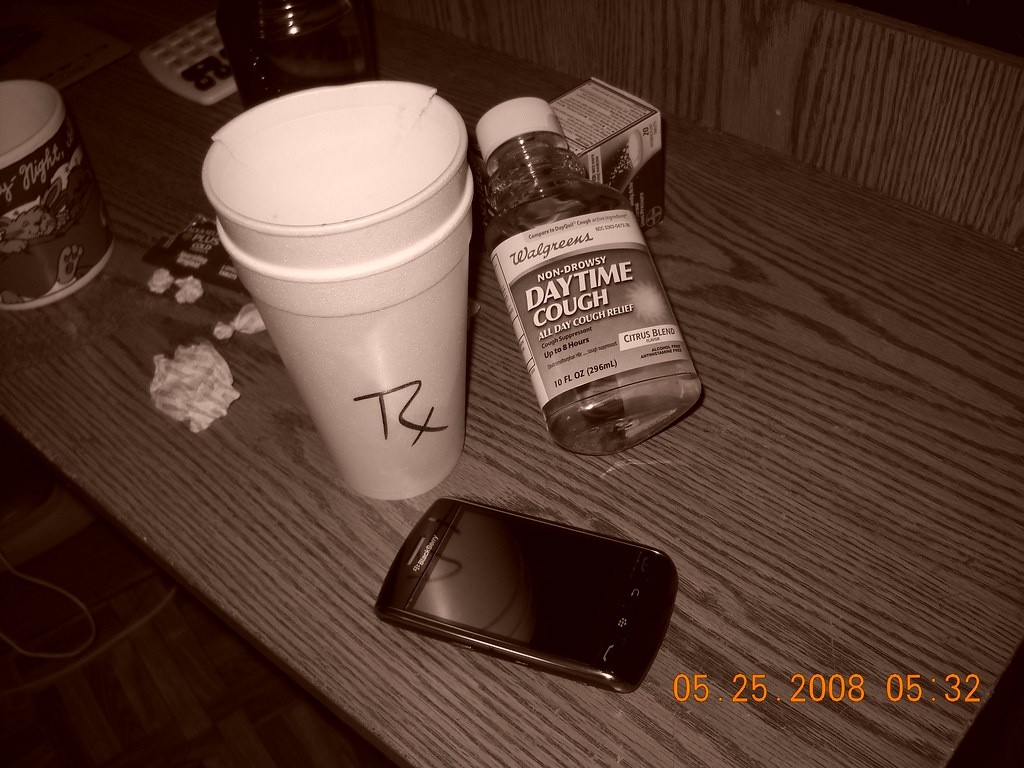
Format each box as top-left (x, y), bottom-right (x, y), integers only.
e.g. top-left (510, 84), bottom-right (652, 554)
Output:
top-left (374, 497), bottom-right (679, 694)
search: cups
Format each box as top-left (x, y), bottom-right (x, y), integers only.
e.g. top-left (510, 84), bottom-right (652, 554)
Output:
top-left (202, 81), bottom-right (474, 500)
top-left (1, 80), bottom-right (114, 311)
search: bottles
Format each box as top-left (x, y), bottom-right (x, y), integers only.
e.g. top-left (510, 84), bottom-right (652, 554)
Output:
top-left (214, 0), bottom-right (381, 117)
top-left (476, 96), bottom-right (702, 455)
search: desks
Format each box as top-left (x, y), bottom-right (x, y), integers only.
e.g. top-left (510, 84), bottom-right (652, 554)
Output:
top-left (1, 1), bottom-right (1023, 768)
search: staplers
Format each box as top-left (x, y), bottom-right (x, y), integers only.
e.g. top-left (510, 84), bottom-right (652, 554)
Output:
top-left (140, 9), bottom-right (239, 106)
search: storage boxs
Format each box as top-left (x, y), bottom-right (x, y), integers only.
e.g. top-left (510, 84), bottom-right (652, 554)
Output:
top-left (550, 75), bottom-right (667, 233)
